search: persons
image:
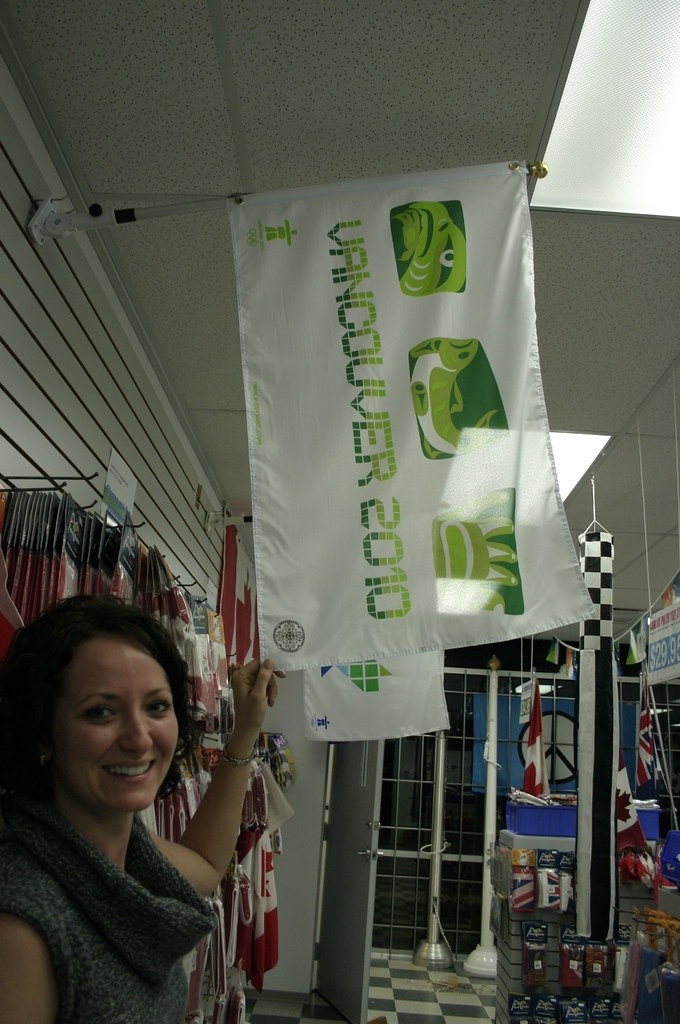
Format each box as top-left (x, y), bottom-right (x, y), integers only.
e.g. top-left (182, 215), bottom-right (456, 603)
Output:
top-left (0, 597), bottom-right (286, 1024)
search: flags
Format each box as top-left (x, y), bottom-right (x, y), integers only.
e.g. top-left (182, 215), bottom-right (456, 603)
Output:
top-left (617, 751), bottom-right (646, 852)
top-left (638, 689), bottom-right (660, 802)
top-left (226, 166), bottom-right (596, 676)
top-left (522, 690), bottom-right (550, 794)
top-left (301, 650), bottom-right (449, 741)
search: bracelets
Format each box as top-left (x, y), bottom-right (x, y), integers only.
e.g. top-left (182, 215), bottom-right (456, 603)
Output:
top-left (223, 743), bottom-right (255, 765)
